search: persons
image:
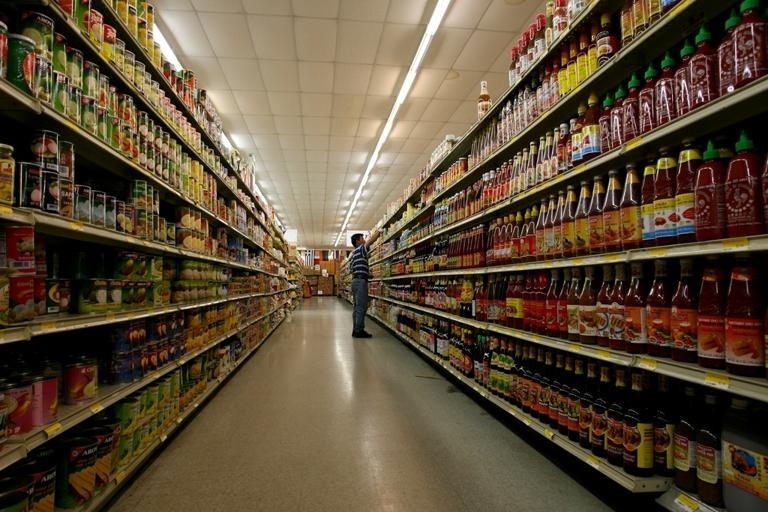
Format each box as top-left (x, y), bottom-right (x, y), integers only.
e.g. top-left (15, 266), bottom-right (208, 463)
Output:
top-left (348, 228), bottom-right (384, 338)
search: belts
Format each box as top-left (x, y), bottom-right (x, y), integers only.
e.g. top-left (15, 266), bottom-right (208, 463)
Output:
top-left (352, 276), bottom-right (367, 279)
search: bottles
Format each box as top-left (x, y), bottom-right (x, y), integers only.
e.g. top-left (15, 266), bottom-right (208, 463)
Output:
top-left (370, 1), bottom-right (768, 512)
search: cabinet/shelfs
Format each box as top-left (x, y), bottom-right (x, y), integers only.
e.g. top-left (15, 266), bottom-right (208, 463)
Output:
top-left (339, 252), bottom-right (353, 306)
top-left (319, 259), bottom-right (336, 275)
top-left (318, 276), bottom-right (334, 295)
top-left (289, 246), bottom-right (304, 310)
top-left (304, 280), bottom-right (312, 298)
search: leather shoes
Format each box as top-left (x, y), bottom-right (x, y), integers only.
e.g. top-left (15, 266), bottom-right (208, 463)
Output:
top-left (353, 330), bottom-right (372, 338)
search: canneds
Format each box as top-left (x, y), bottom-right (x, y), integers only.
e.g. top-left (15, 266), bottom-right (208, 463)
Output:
top-left (0, 318), bottom-right (198, 512)
top-left (163, 60), bottom-right (246, 228)
top-left (181, 304), bottom-right (250, 412)
top-left (0, 124), bottom-right (164, 327)
top-left (0, 1), bottom-right (167, 185)
top-left (162, 230), bottom-right (260, 307)
